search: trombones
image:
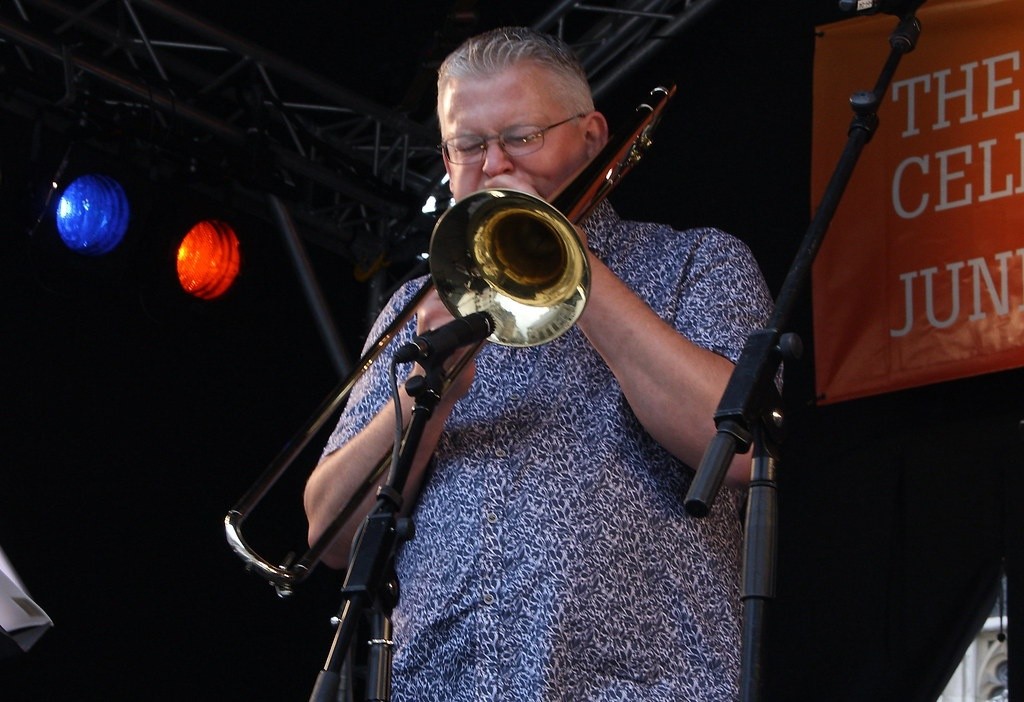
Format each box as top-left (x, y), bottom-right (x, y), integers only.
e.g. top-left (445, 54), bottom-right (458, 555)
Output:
top-left (223, 81), bottom-right (685, 601)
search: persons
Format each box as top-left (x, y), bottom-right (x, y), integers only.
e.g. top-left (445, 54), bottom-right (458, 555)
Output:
top-left (303, 26), bottom-right (783, 702)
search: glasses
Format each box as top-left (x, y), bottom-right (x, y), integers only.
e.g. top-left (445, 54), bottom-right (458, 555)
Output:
top-left (436, 113), bottom-right (586, 166)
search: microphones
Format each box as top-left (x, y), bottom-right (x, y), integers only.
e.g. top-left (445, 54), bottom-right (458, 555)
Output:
top-left (838, 0), bottom-right (923, 14)
top-left (394, 310), bottom-right (497, 363)
